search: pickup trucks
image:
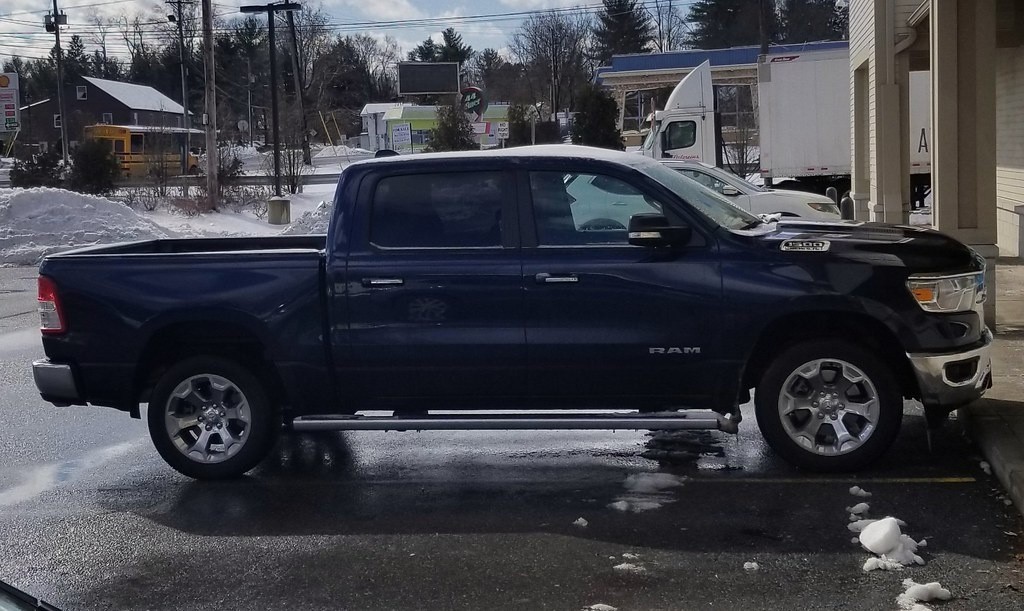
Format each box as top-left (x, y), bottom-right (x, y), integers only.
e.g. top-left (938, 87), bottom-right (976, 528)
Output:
top-left (28, 140), bottom-right (997, 482)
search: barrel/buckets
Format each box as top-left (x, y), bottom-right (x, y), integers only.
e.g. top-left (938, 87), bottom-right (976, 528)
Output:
top-left (267, 199), bottom-right (291, 225)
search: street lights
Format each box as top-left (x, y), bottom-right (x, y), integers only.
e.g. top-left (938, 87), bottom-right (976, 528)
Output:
top-left (239, 3), bottom-right (303, 197)
top-left (24, 93), bottom-right (34, 163)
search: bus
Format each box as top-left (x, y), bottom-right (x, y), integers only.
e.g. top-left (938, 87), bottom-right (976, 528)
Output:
top-left (82, 121), bottom-right (206, 181)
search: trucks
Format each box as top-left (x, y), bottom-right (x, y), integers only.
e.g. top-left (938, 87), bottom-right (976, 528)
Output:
top-left (638, 47), bottom-right (934, 208)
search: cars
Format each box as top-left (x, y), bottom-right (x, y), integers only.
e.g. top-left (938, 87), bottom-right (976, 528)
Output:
top-left (562, 153), bottom-right (842, 229)
